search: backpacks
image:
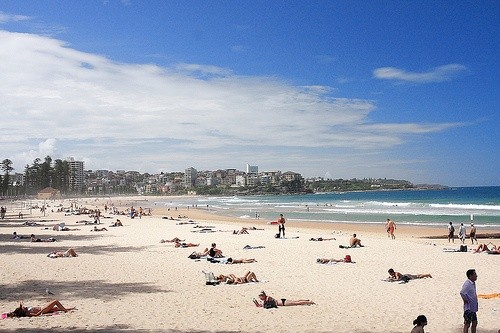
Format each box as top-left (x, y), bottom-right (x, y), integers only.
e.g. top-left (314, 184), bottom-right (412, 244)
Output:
top-left (449, 225), bottom-right (454, 232)
top-left (344, 255), bottom-right (351, 263)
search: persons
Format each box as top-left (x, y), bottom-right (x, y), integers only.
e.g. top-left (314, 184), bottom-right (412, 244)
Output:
top-left (448, 222), bottom-right (466, 244)
top-left (469, 223), bottom-right (477, 244)
top-left (256, 211), bottom-right (260, 220)
top-left (305, 204), bottom-right (309, 212)
top-left (161, 237), bottom-right (258, 264)
top-left (311, 237), bottom-right (336, 241)
top-left (256, 290), bottom-right (315, 307)
top-left (460, 269), bottom-right (478, 333)
top-left (349, 233), bottom-right (364, 248)
top-left (317, 258), bottom-right (343, 264)
top-left (385, 268), bottom-right (432, 281)
top-left (48, 248), bottom-right (78, 258)
top-left (473, 242), bottom-right (500, 254)
top-left (411, 315), bottom-right (427, 333)
top-left (217, 270), bottom-right (259, 285)
top-left (0, 203), bottom-right (151, 242)
top-left (277, 214), bottom-right (286, 238)
top-left (168, 203), bottom-right (216, 228)
top-left (233, 225), bottom-right (265, 234)
top-left (14, 299), bottom-right (79, 316)
top-left (384, 218), bottom-right (396, 239)
